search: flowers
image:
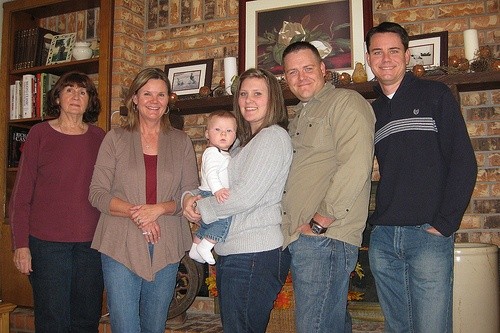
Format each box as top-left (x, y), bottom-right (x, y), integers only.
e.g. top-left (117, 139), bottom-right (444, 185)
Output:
top-left (205, 273), bottom-right (218, 296)
top-left (273, 272), bottom-right (292, 310)
top-left (346, 262), bottom-right (365, 303)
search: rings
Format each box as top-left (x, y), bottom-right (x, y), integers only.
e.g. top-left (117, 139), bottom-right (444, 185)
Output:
top-left (142, 232), bottom-right (147, 235)
top-left (148, 232), bottom-right (151, 235)
top-left (137, 218), bottom-right (140, 223)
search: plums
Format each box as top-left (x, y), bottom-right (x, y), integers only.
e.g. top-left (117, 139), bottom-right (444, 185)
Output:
top-left (448, 56), bottom-right (458, 66)
top-left (199, 85), bottom-right (210, 96)
top-left (480, 47), bottom-right (490, 57)
top-left (169, 93), bottom-right (177, 101)
top-left (413, 64), bottom-right (424, 77)
top-left (457, 58), bottom-right (469, 70)
top-left (339, 72), bottom-right (351, 85)
top-left (219, 78), bottom-right (225, 86)
top-left (491, 59), bottom-right (500, 72)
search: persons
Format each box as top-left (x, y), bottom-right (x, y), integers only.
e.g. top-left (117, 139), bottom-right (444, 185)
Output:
top-left (10, 73), bottom-right (105, 333)
top-left (366, 22), bottom-right (477, 333)
top-left (181, 69), bottom-right (293, 333)
top-left (89, 68), bottom-right (198, 332)
top-left (189, 110), bottom-right (237, 265)
top-left (282, 41), bottom-right (376, 332)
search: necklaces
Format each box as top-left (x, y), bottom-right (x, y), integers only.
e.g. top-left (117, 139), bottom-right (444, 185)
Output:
top-left (145, 143), bottom-right (150, 150)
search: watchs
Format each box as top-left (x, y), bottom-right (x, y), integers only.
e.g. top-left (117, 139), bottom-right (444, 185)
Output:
top-left (309, 218), bottom-right (327, 235)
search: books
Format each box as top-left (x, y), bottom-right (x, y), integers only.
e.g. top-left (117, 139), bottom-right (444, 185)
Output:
top-left (6, 27), bottom-right (60, 168)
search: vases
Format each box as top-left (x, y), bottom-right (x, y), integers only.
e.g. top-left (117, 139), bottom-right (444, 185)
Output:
top-left (454, 242), bottom-right (500, 332)
top-left (214, 296), bottom-right (220, 314)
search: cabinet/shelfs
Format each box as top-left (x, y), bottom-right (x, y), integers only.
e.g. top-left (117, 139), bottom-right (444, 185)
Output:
top-left (0, 0), bottom-right (115, 316)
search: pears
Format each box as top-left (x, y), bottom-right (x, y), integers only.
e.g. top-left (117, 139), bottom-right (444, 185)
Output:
top-left (352, 62), bottom-right (367, 83)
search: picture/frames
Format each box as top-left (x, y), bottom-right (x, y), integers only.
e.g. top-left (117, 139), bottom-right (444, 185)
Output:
top-left (238, 0), bottom-right (373, 83)
top-left (46, 33), bottom-right (76, 66)
top-left (164, 58), bottom-right (214, 99)
top-left (408, 30), bottom-right (448, 72)
